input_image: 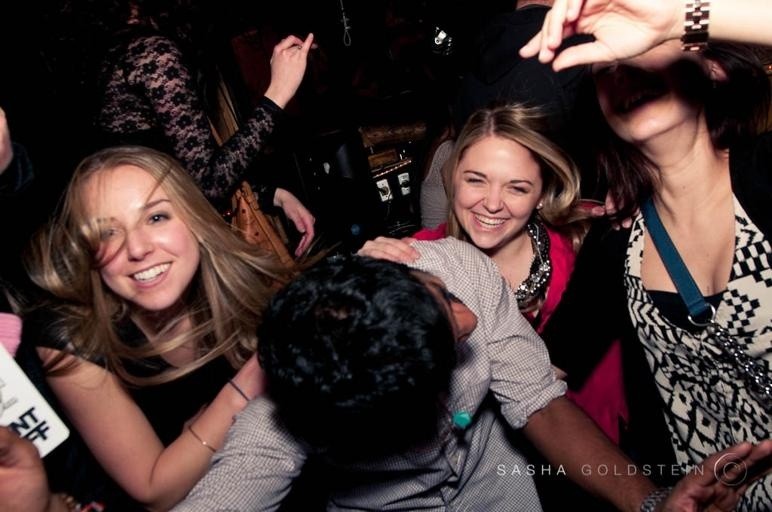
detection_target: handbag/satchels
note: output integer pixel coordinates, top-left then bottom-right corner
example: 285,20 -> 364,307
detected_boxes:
231,182 -> 295,293
